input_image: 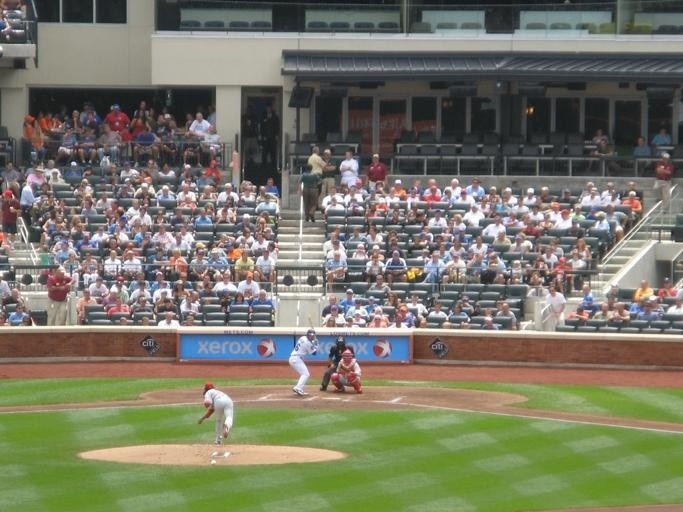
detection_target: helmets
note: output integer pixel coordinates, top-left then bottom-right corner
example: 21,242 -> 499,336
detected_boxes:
342,349 -> 352,363
336,336 -> 345,348
307,329 -> 315,336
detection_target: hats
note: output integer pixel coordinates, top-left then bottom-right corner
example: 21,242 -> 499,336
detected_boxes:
205,383 -> 213,389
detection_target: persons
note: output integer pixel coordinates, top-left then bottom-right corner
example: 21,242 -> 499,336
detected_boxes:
288,328 -> 320,395
197,382 -> 236,446
0,95 -> 280,326
300,124 -> 683,331
0,9 -> 12,43
319,335 -> 355,390
330,348 -> 363,394
0,0 -> 22,10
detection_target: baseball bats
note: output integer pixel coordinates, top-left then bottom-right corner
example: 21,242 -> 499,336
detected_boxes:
308,317 -> 317,339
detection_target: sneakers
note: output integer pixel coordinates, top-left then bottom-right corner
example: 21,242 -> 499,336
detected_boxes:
320,384 -> 327,391
214,424 -> 228,444
334,386 -> 344,392
354,387 -> 362,393
292,388 -> 304,396
